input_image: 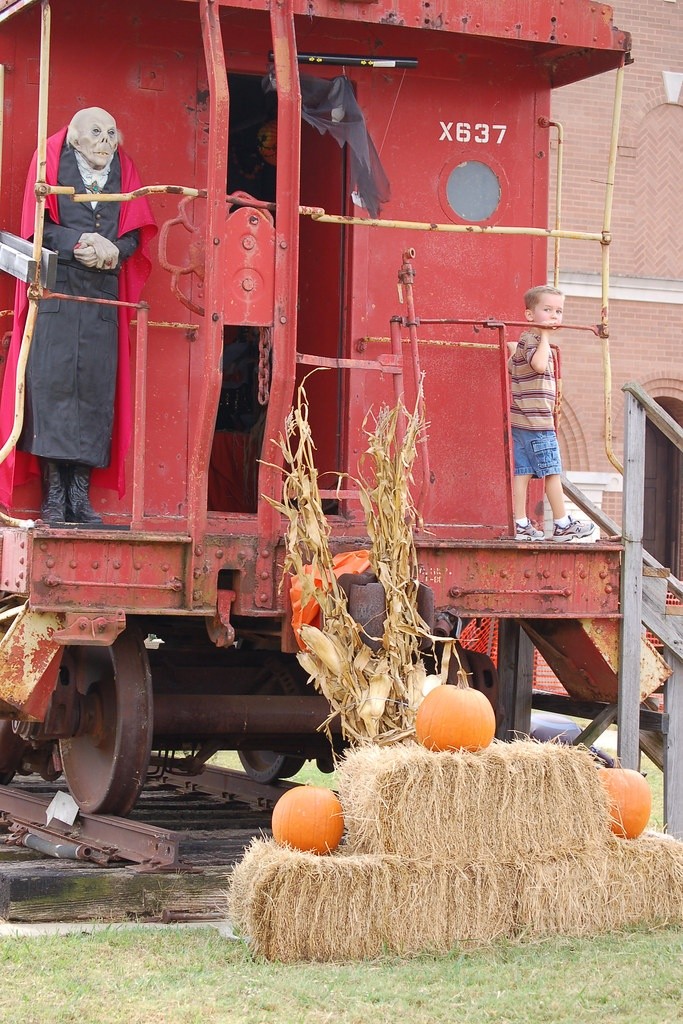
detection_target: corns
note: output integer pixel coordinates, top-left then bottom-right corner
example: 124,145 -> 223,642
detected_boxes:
296,623 -> 341,681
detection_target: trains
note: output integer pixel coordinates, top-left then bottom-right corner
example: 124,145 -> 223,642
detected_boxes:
0,1 -> 675,820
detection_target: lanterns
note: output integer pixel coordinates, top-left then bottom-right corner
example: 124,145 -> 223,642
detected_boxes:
256,121 -> 278,166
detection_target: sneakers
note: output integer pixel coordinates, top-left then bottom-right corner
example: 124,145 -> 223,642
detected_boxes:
515,519 -> 545,541
552,515 -> 595,542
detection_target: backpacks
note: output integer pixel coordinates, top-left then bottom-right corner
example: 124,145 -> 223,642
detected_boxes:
213,330 -> 263,433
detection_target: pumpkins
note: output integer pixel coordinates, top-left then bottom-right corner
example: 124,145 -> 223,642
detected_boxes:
415,672 -> 495,754
272,781 -> 344,856
596,757 -> 652,839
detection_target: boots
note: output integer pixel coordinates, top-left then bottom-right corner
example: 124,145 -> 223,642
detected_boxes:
67,462 -> 102,522
41,458 -> 68,522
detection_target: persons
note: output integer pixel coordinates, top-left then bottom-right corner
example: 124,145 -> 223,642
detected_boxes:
508,286 -> 596,540
0,106 -> 160,524
